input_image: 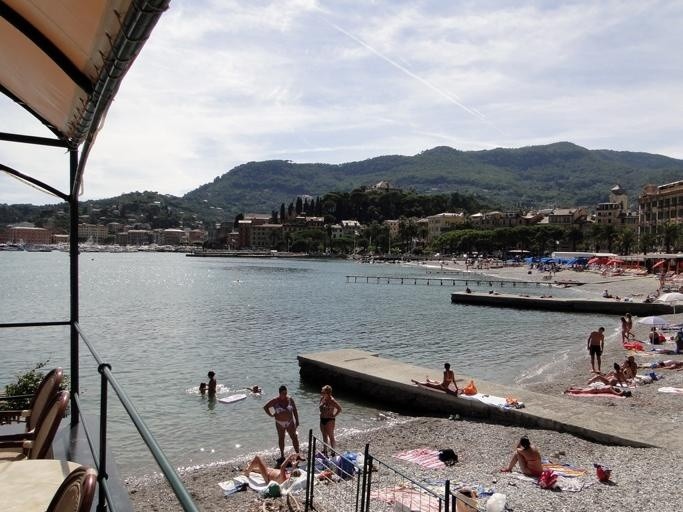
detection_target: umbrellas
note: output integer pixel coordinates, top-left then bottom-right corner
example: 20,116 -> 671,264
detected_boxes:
651,291 -> 683,319
525,256 -> 624,272
637,316 -> 670,344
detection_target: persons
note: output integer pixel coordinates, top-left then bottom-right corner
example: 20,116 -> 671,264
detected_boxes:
199,371 -> 216,399
651,289 -> 660,297
637,359 -> 683,369
620,313 -> 635,343
649,326 -> 659,344
319,385 -> 342,457
263,386 -> 305,461
250,385 -> 261,393
241,452 -> 301,485
602,289 -> 612,298
501,436 -> 542,476
563,356 -> 637,396
411,362 -> 458,393
587,327 -> 605,373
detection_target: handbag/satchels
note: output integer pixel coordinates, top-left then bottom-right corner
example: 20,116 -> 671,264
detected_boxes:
439,448 -> 458,466
537,469 -> 558,489
463,381 -> 477,395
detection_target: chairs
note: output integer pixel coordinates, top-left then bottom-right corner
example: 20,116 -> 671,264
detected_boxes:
45,463 -> 98,512
0,367 -> 71,457
